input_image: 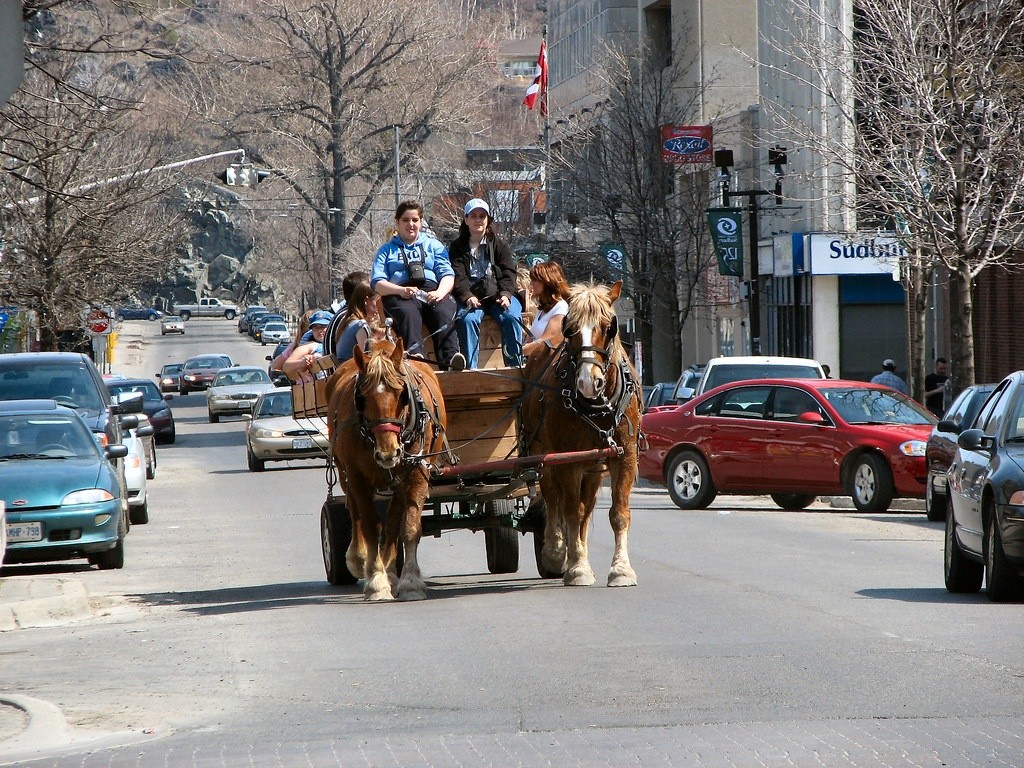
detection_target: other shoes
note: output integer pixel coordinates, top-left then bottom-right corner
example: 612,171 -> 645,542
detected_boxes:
448,353 -> 466,371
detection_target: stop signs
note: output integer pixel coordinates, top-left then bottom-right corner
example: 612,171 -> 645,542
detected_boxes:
87,309 -> 109,332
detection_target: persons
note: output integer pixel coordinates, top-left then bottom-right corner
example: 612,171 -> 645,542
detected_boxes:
449,198 -> 522,370
370,200 -> 466,370
225,375 -> 233,386
870,357 -> 952,420
522,261 -> 573,356
822,365 -> 832,379
253,372 -> 261,382
268,397 -> 284,413
271,271 -> 391,381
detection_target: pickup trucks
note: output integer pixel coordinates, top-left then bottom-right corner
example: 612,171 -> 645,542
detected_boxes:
172,296 -> 241,320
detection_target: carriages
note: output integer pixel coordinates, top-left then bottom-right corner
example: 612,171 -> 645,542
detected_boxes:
319,279 -> 641,603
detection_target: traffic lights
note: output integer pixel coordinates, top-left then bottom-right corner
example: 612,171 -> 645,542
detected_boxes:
213,168 -> 227,185
258,169 -> 270,184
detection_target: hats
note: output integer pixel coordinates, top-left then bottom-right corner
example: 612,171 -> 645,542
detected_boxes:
464,198 -> 489,216
882,358 -> 896,366
307,310 -> 335,329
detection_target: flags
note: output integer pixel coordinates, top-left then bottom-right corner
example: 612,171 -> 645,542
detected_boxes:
522,41 -> 546,119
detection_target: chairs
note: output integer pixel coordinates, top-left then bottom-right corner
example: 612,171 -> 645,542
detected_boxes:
48,376 -> 78,400
191,362 -> 199,369
223,375 -> 233,385
250,373 -> 261,382
722,404 -> 764,415
35,429 -> 75,454
211,362 -> 219,368
111,387 -> 150,400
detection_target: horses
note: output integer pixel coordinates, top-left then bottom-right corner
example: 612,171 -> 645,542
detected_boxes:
323,336 -> 448,603
524,278 -> 644,586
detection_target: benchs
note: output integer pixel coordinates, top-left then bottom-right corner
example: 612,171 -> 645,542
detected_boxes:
374,297 -> 534,328
283,354 -> 338,418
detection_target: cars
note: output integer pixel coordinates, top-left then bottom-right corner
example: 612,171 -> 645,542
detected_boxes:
161,316 -> 186,335
264,341 -> 298,386
1,352 -> 157,572
637,351 -> 1004,522
938,367 -> 1024,602
637,378 -> 940,513
242,386 -> 332,471
237,303 -> 291,346
206,366 -> 282,423
104,379 -> 176,444
155,363 -> 184,393
114,304 -> 164,321
181,355 -> 240,395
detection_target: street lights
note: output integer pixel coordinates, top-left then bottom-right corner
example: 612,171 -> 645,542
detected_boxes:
532,193 -> 651,385
715,143 -> 788,356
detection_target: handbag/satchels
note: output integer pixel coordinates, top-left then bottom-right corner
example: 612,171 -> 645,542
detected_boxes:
470,273 -> 498,306
408,261 -> 426,283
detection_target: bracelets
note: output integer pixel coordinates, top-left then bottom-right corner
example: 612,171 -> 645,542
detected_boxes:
534,339 -> 541,346
